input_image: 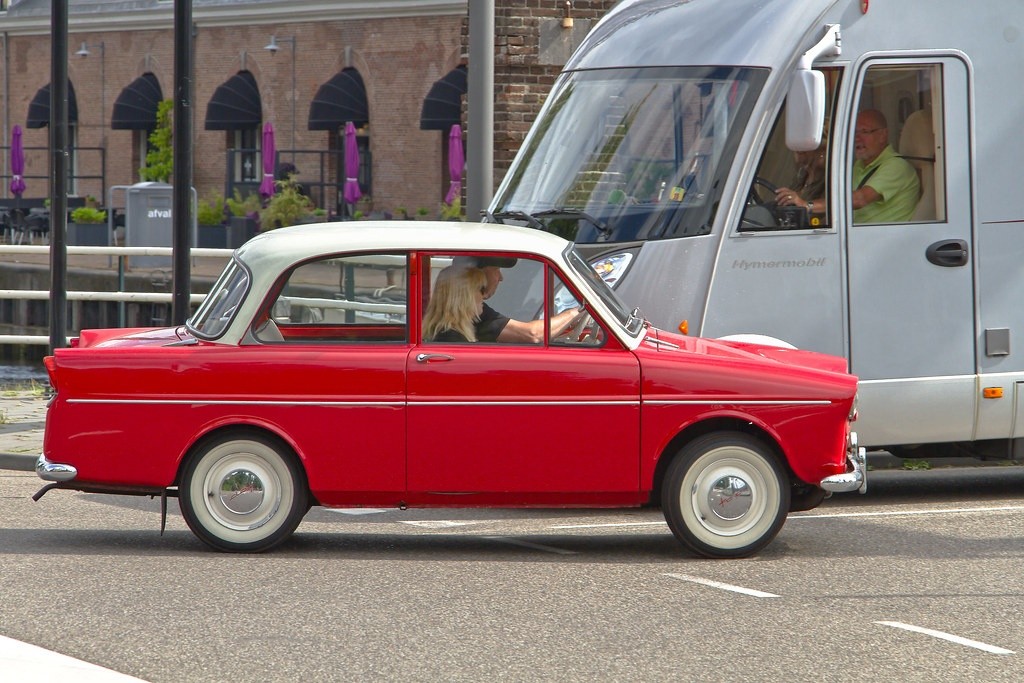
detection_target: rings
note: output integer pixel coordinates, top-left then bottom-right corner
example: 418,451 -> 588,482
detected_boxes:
787,195 -> 792,199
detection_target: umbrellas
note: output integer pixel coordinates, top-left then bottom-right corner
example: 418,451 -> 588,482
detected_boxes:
260,121 -> 274,196
10,124 -> 26,200
444,123 -> 464,206
345,120 -> 361,219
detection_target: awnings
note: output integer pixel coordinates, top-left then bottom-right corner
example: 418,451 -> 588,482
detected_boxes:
110,72 -> 164,130
418,63 -> 468,130
308,67 -> 368,131
204,70 -> 262,130
26,77 -> 78,129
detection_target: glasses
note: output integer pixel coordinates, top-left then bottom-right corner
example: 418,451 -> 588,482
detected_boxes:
480,285 -> 486,296
856,128 -> 881,134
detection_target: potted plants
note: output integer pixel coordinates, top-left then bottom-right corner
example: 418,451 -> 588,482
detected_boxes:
312,207 -> 328,223
67,206 -> 108,247
225,188 -> 263,249
354,210 -> 364,221
391,207 -> 408,220
356,195 -> 375,211
197,186 -> 231,250
414,207 -> 430,221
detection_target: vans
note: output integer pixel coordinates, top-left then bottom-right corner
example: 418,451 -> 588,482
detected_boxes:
477,0 -> 1024,463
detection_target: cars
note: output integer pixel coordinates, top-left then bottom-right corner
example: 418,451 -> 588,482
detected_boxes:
33,224 -> 867,555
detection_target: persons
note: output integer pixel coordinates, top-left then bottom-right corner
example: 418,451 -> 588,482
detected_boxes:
421,256 -> 593,344
774,110 -> 920,227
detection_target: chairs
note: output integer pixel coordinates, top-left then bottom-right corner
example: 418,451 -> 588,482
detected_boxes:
897,109 -> 937,221
0,206 -> 124,247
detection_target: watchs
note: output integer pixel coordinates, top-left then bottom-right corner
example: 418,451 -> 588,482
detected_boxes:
808,200 -> 813,214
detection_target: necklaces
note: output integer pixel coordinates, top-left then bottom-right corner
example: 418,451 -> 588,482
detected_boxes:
805,180 -> 814,187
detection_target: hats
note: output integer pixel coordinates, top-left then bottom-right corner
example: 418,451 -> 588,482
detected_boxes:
452,255 -> 517,269
